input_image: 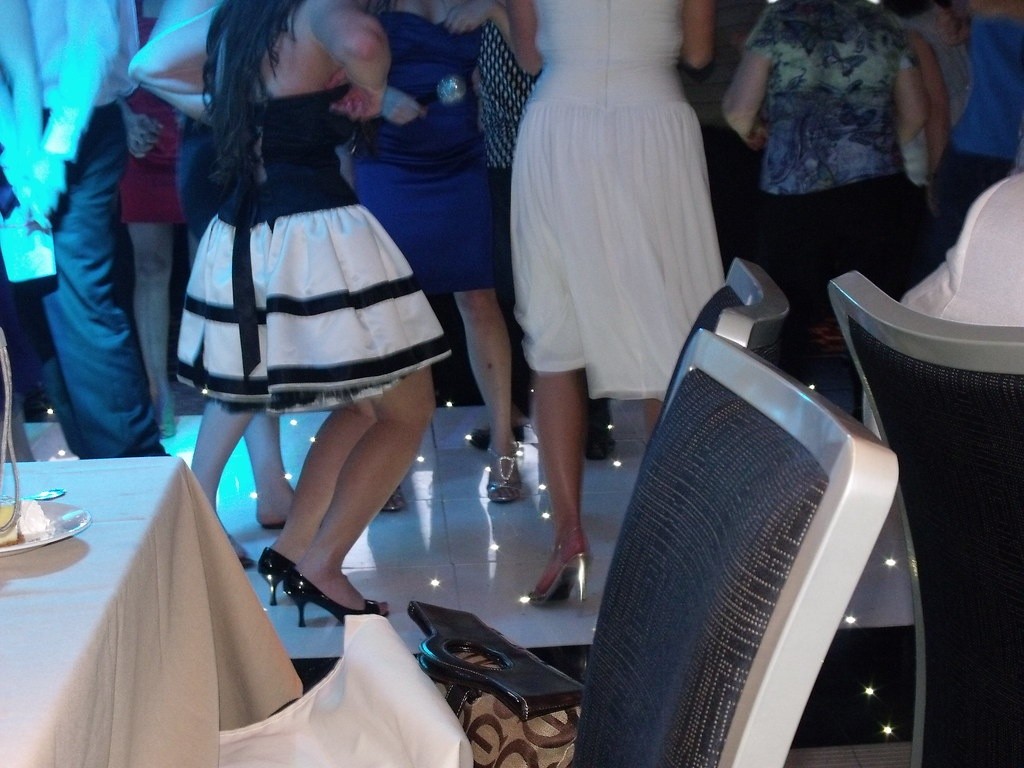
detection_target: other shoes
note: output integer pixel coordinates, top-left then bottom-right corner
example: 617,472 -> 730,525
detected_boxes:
584,425 -> 610,460
470,427 -> 526,448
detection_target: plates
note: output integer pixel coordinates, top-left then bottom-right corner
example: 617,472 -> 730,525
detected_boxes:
0,501 -> 92,557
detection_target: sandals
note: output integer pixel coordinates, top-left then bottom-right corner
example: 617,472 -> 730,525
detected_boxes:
487,443 -> 522,501
381,487 -> 404,512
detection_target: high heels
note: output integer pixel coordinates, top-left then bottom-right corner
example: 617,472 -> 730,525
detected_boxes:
257,547 -> 297,606
283,567 -> 389,628
529,525 -> 591,605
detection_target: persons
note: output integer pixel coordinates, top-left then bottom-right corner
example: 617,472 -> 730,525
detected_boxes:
116,0 -> 181,439
467,0 -> 612,460
1,0 -> 172,457
179,406 -> 292,569
128,0 -> 452,627
350,0 -> 522,504
720,0 -> 1024,422
508,1 -> 725,604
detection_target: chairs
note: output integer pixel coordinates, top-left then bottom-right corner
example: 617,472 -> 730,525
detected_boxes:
640,258 -> 790,465
829,269 -> 1024,768
409,328 -> 899,768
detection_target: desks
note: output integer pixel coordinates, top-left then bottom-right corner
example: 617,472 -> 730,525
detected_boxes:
0,454 -> 303,768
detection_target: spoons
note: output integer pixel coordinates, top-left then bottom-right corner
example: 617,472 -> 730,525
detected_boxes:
20,488 -> 66,500
